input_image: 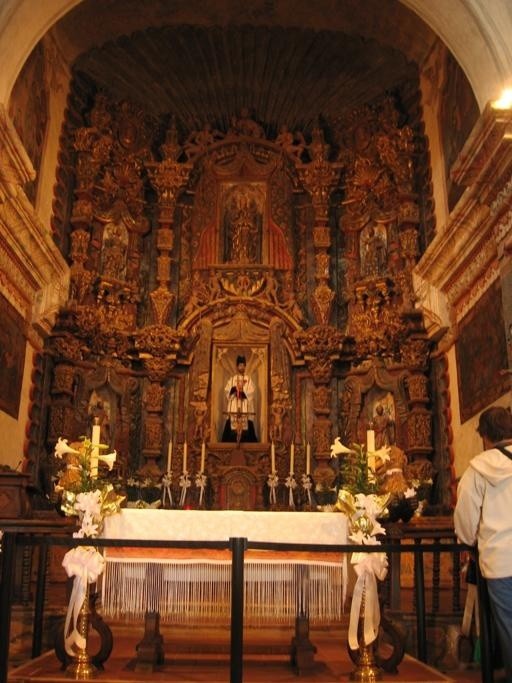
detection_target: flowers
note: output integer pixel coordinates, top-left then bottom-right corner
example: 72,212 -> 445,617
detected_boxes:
324,435 -> 435,497
47,431 -> 128,492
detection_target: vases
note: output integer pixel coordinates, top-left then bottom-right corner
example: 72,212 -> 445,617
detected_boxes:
336,489 -> 390,681
59,482 -> 126,682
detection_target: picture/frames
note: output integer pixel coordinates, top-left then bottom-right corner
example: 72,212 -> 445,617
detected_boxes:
208,338 -> 272,450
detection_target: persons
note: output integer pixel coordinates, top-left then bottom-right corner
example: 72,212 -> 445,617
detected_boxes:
454,406 -> 512,683
182,118 -> 303,159
102,228 -> 128,278
221,354 -> 258,443
194,408 -> 207,438
271,405 -> 286,438
373,405 -> 389,448
85,401 -> 110,444
363,226 -> 384,277
176,269 -> 299,324
224,194 -> 257,262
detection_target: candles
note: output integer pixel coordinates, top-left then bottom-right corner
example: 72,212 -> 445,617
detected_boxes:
167,438 -> 173,474
200,440 -> 206,475
182,438 -> 188,473
91,425 -> 100,485
271,439 -> 277,474
289,440 -> 294,475
305,440 -> 311,476
366,429 -> 377,485
